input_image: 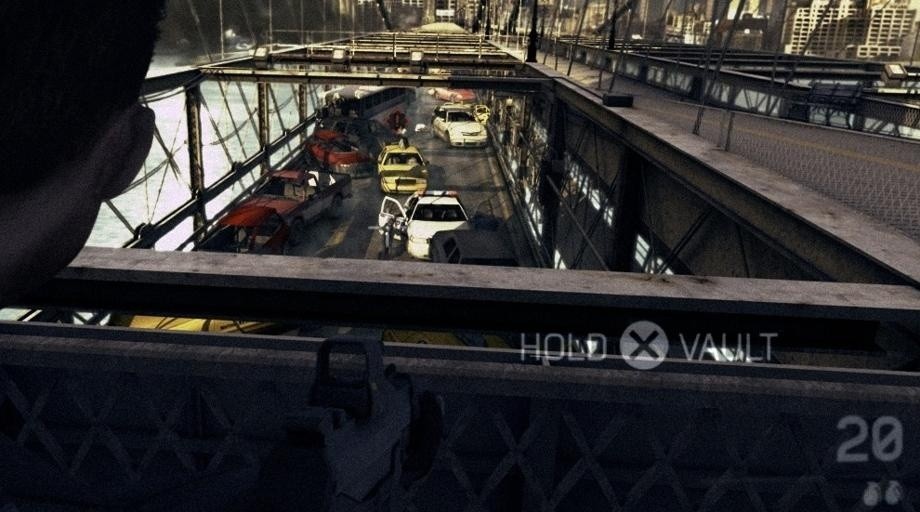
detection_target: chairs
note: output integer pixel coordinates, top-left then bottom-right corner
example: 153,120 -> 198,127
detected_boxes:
442,209 -> 457,219
386,158 -> 397,164
406,157 -> 417,165
421,209 -> 433,219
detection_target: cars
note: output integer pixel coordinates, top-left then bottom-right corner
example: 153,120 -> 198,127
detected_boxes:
378,138 -> 429,194
378,189 -> 474,261
430,88 -> 490,151
127,312 -> 274,336
428,229 -> 520,266
189,206 -> 292,256
307,116 -> 398,178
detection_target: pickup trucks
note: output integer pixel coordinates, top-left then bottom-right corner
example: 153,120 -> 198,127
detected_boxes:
239,169 -> 354,236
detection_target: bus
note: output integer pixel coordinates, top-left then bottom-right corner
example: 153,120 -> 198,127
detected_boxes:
315,86 -> 418,135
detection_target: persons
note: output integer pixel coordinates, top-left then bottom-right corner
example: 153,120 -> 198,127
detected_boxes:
396,124 -> 408,148
377,215 -> 395,259
1,0 -> 169,307
